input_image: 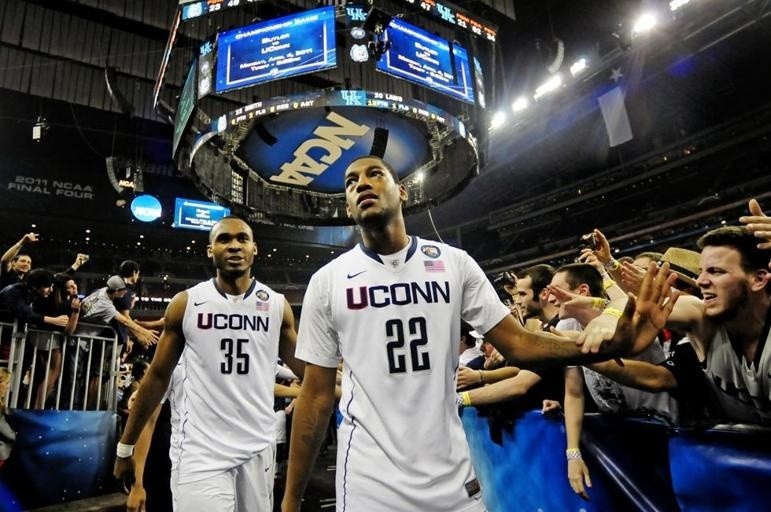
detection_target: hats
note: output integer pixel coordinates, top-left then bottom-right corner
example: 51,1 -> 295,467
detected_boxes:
644,246 -> 701,287
106,275 -> 134,290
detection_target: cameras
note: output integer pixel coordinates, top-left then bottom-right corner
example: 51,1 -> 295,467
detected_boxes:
493,271 -> 515,286
71,295 -> 99,313
578,233 -> 595,250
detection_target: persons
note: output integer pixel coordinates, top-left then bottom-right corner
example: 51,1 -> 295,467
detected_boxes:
114,217 -> 305,512
456,155 -> 770,507
0,232 -> 164,417
275,357 -> 344,490
283,155 -> 679,512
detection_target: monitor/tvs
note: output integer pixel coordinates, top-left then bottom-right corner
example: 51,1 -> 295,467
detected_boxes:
172,197 -> 231,233
